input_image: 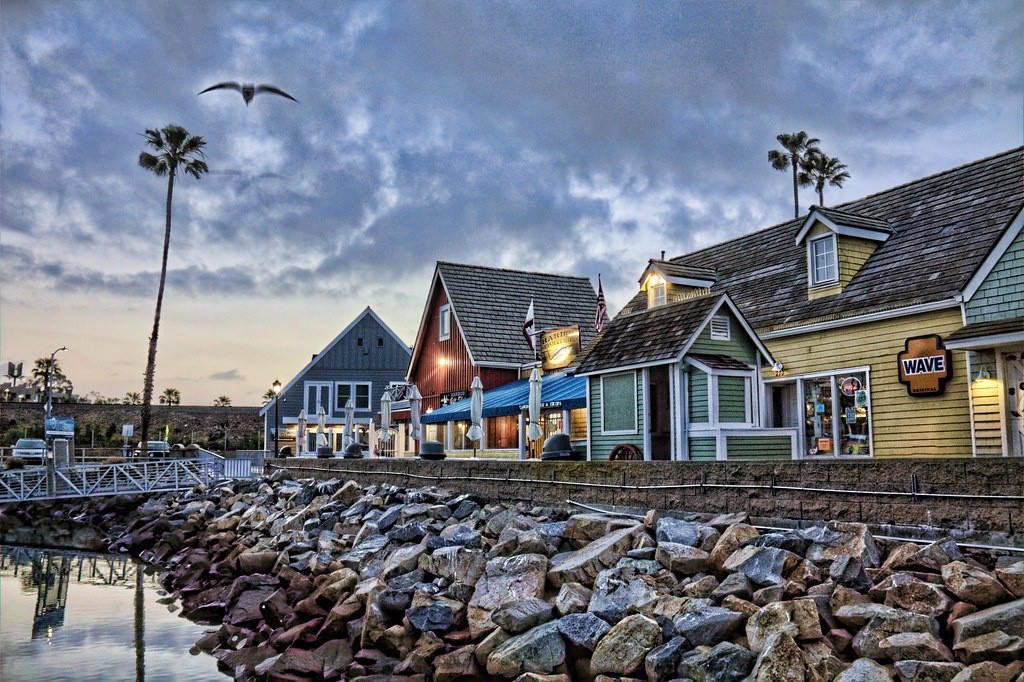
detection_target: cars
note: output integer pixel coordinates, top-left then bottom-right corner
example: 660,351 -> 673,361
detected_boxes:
11,438 -> 48,465
134,440 -> 172,466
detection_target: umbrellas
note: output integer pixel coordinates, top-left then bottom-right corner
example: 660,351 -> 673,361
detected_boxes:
527,364 -> 544,459
376,427 -> 397,454
343,399 -> 355,455
296,409 -> 307,457
407,385 -> 424,458
377,391 -> 391,458
466,375 -> 485,458
316,407 -> 327,455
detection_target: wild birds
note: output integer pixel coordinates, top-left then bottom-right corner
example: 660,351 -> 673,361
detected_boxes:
196,79 -> 303,108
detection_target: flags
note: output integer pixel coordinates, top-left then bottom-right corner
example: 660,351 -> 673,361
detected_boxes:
522,299 -> 536,360
593,277 -> 607,333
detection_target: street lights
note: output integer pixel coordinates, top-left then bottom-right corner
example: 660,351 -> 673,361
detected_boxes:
46,346 -> 69,417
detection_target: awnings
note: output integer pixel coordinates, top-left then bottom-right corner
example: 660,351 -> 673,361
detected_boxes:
419,372 -> 586,424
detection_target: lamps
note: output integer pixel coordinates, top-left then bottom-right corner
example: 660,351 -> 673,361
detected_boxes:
974,351 -> 990,381
